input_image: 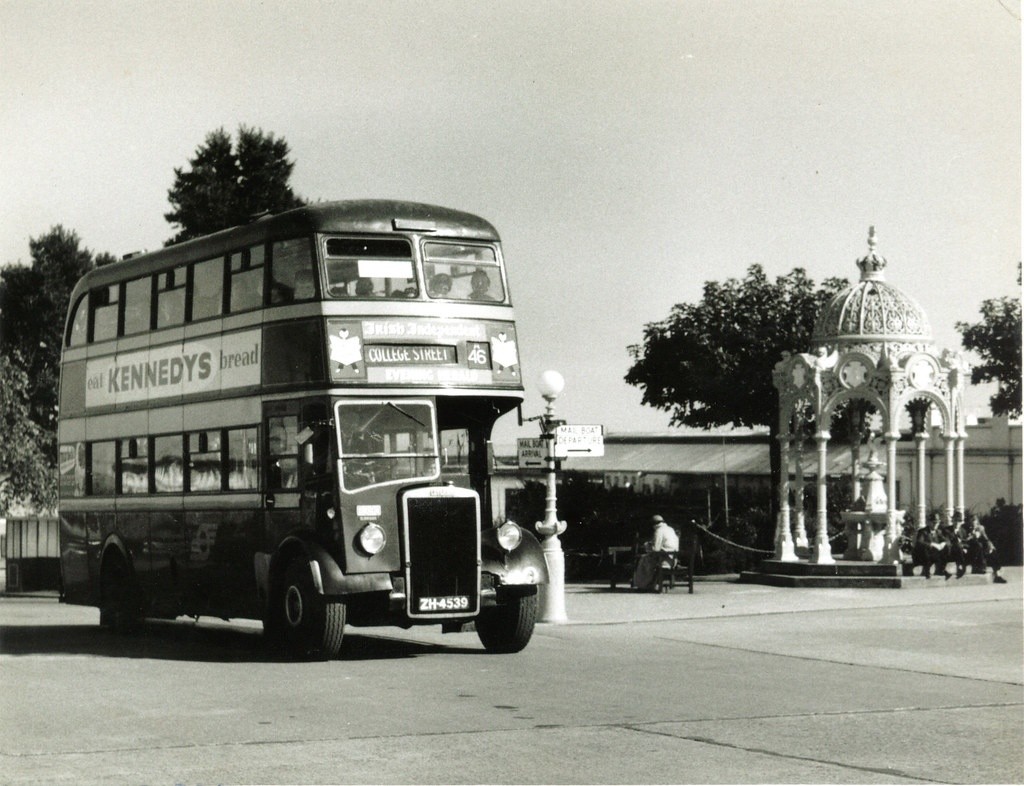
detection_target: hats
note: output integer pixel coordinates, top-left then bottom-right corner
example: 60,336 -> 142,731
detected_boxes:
650,515 -> 665,526
928,512 -> 941,523
951,511 -> 962,522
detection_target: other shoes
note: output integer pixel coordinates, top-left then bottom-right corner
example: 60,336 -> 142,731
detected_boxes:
935,570 -> 949,575
973,567 -> 986,574
993,576 -> 1006,583
958,569 -> 964,575
922,570 -> 930,576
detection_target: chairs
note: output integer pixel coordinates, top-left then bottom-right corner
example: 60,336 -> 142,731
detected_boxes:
650,550 -> 696,594
607,546 -> 633,590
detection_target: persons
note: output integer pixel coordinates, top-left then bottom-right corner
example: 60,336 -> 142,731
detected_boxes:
956,515 -> 1008,583
913,512 -> 965,579
628,511 -> 681,592
